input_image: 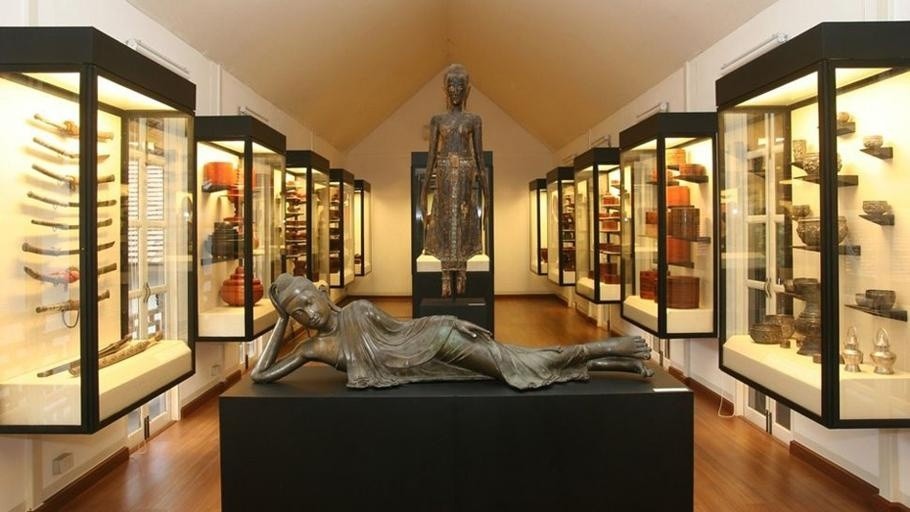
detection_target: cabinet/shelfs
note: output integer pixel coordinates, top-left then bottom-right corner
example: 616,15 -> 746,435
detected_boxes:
196,116 -> 286,343
284,150 -> 330,297
353,179 -> 371,277
620,112 -> 717,338
411,152 -> 494,340
330,169 -> 355,289
529,178 -> 547,276
546,166 -> 575,287
1,25 -> 197,435
574,148 -> 621,304
218,366 -> 694,511
715,21 -> 910,429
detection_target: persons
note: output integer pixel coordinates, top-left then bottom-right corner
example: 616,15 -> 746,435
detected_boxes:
419,63 -> 491,297
250,272 -> 656,390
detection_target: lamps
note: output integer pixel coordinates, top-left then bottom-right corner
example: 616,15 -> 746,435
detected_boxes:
240,106 -> 269,127
584,135 -> 610,151
127,37 -> 190,79
720,32 -> 786,77
636,102 -> 668,125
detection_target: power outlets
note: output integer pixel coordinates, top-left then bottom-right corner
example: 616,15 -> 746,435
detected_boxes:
52,453 -> 73,476
